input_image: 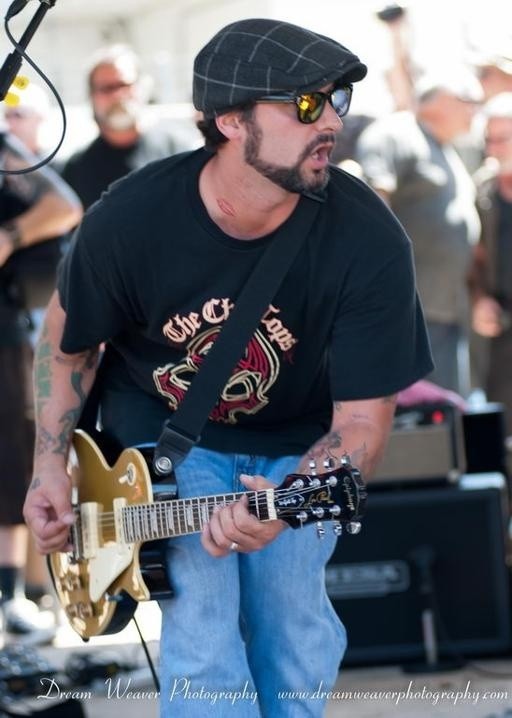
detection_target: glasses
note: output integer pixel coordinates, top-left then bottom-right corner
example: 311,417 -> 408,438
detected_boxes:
257,84 -> 353,123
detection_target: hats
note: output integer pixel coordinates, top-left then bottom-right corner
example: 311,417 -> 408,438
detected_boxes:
193,19 -> 367,119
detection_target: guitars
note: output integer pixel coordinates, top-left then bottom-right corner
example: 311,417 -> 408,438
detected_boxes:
47,426 -> 368,642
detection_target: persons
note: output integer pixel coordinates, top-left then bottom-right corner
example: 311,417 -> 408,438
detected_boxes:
0,1 -> 512,718
